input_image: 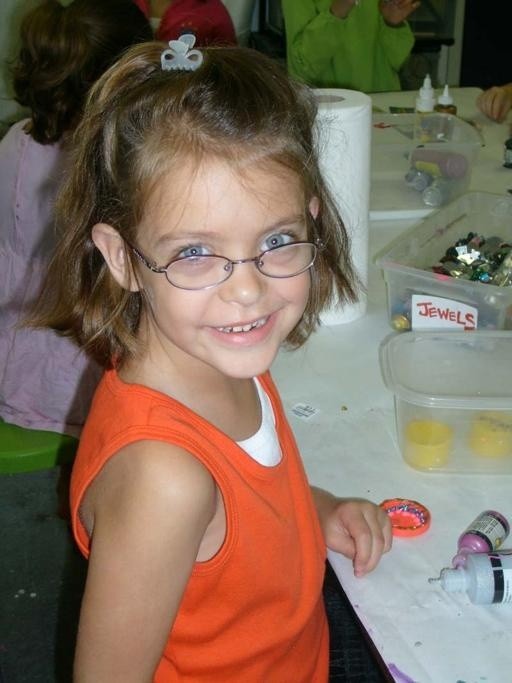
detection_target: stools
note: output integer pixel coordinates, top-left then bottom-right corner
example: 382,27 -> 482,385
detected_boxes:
0,416 -> 79,480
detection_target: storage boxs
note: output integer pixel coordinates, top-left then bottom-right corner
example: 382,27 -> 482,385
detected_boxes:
374,190 -> 511,329
380,333 -> 509,476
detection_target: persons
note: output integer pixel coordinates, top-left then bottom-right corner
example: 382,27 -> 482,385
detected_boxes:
147,0 -> 266,52
2,1 -> 156,439
278,1 -> 430,97
23,33 -> 393,680
478,82 -> 508,120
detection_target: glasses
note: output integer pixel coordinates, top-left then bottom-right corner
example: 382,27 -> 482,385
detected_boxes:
119,209 -> 327,290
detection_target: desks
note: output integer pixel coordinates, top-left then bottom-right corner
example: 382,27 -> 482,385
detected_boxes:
260,69 -> 509,683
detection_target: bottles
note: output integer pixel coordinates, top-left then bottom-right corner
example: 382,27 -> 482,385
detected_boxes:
449,508 -> 508,568
426,550 -> 512,607
412,75 -> 458,142
